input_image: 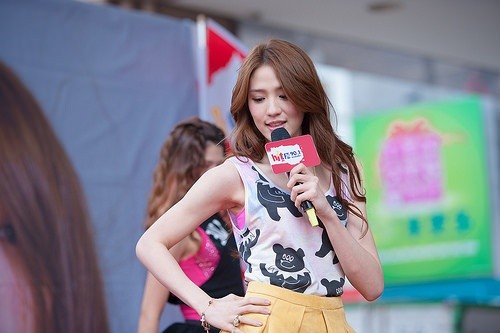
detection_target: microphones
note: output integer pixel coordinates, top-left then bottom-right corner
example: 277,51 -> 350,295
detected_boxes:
264,127 -> 320,227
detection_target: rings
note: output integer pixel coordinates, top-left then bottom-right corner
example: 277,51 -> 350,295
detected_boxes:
233,315 -> 240,327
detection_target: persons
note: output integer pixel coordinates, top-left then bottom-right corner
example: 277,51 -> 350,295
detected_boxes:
0,63 -> 110,333
137,120 -> 246,333
136,39 -> 384,333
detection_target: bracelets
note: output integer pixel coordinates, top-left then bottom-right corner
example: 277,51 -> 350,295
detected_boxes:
200,298 -> 214,333
